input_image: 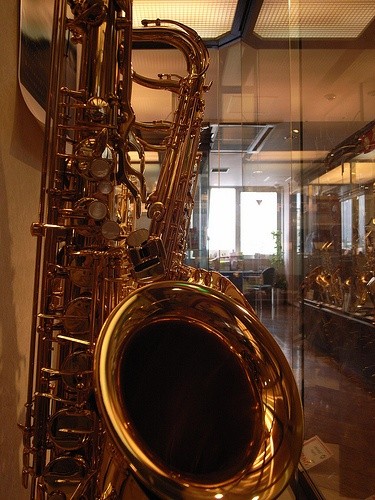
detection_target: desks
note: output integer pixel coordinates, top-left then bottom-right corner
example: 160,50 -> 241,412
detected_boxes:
211,268 -> 262,311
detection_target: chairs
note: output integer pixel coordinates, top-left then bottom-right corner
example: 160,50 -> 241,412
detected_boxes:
253,266 -> 278,318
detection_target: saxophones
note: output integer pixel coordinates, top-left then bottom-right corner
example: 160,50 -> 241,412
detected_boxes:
15,0 -> 304,500
317,226 -> 375,304
